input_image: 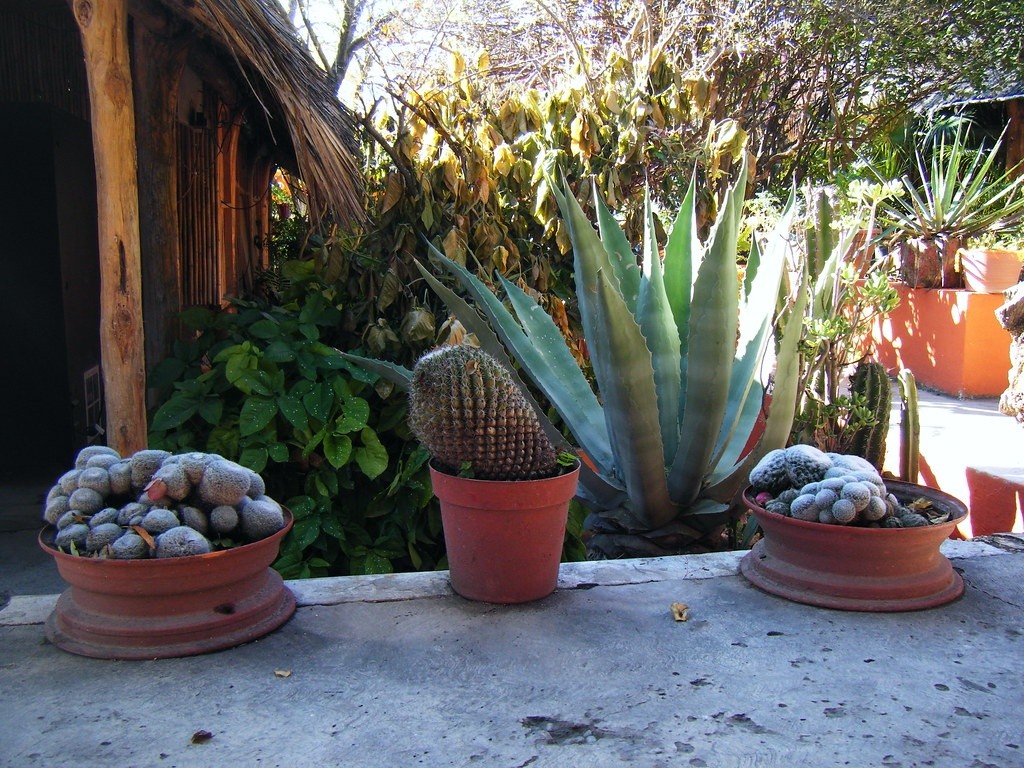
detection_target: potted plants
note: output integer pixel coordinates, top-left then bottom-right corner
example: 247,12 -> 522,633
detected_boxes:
955,232 -> 1024,290
37,443 -> 298,660
833,181 -> 904,278
741,447 -> 971,613
845,114 -> 1024,288
407,348 -> 583,601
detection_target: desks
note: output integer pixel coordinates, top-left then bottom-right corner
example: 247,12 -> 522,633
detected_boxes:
845,281 -> 1013,400
